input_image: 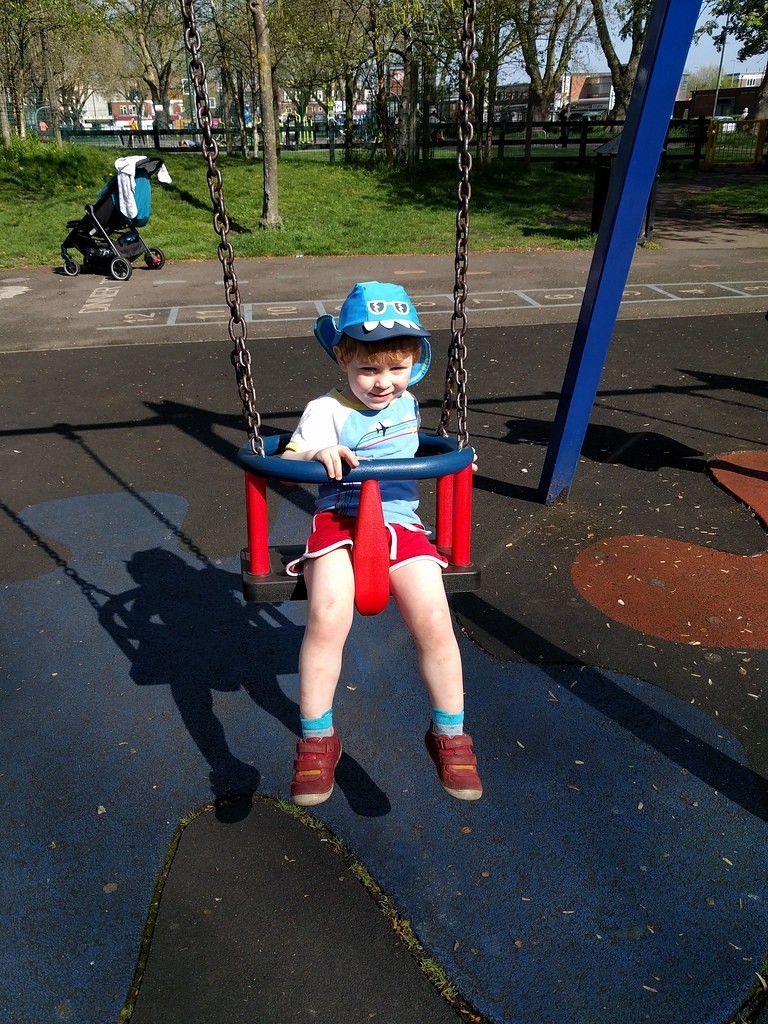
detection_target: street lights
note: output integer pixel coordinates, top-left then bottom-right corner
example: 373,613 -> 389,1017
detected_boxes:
731,59 -> 736,88
180,40 -> 196,142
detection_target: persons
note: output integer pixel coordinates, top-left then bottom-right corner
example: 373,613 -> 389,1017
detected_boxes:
276,282 -> 482,805
39,120 -> 48,140
179,140 -> 207,147
127,118 -> 137,147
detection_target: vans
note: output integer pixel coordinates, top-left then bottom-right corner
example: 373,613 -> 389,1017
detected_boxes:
708,116 -> 736,134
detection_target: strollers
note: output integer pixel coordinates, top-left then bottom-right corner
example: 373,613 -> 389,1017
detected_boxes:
60,156 -> 164,281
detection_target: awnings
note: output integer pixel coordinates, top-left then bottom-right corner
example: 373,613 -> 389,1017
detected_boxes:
582,110 -> 606,116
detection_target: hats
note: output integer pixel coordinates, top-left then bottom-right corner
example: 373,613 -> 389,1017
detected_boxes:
311,280 -> 432,387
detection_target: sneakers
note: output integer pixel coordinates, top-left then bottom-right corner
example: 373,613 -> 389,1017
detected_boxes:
290,722 -> 343,805
424,716 -> 483,801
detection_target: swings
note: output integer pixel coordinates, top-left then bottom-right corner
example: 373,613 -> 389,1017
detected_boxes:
178,0 -> 481,605
